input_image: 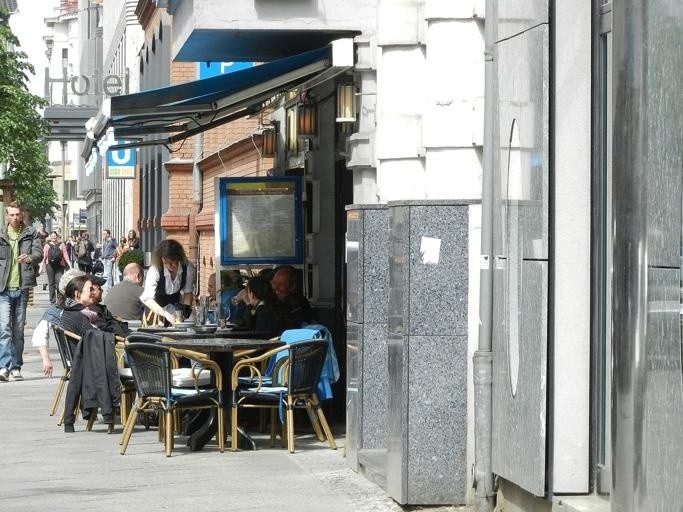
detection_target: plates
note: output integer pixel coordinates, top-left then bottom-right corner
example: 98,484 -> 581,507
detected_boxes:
175,322 -> 195,328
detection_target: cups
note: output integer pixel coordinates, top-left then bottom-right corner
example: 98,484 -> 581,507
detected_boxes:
176,296 -> 234,327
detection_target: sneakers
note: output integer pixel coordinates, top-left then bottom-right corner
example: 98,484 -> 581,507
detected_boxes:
10,369 -> 24,380
0,367 -> 10,381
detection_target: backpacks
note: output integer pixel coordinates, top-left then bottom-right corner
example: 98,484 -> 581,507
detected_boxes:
46,242 -> 62,265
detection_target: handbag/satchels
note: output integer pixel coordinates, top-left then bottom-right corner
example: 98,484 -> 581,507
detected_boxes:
78,244 -> 86,258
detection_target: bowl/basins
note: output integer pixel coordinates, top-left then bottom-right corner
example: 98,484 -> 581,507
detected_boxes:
192,325 -> 218,334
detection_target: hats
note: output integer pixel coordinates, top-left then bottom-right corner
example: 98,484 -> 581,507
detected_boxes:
87,274 -> 106,285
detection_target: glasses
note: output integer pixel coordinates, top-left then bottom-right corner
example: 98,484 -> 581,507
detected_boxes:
81,287 -> 94,292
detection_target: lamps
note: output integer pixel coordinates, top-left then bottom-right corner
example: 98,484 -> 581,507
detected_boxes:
260,129 -> 278,158
335,79 -> 357,123
297,96 -> 317,140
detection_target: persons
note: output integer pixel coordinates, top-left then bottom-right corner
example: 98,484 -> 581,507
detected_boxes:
31,264 -> 314,380
36,220 -> 141,306
105,262 -> 145,320
0,202 -> 45,382
138,239 -> 197,329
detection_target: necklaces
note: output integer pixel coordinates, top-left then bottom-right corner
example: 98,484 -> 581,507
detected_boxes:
7,223 -> 23,241
